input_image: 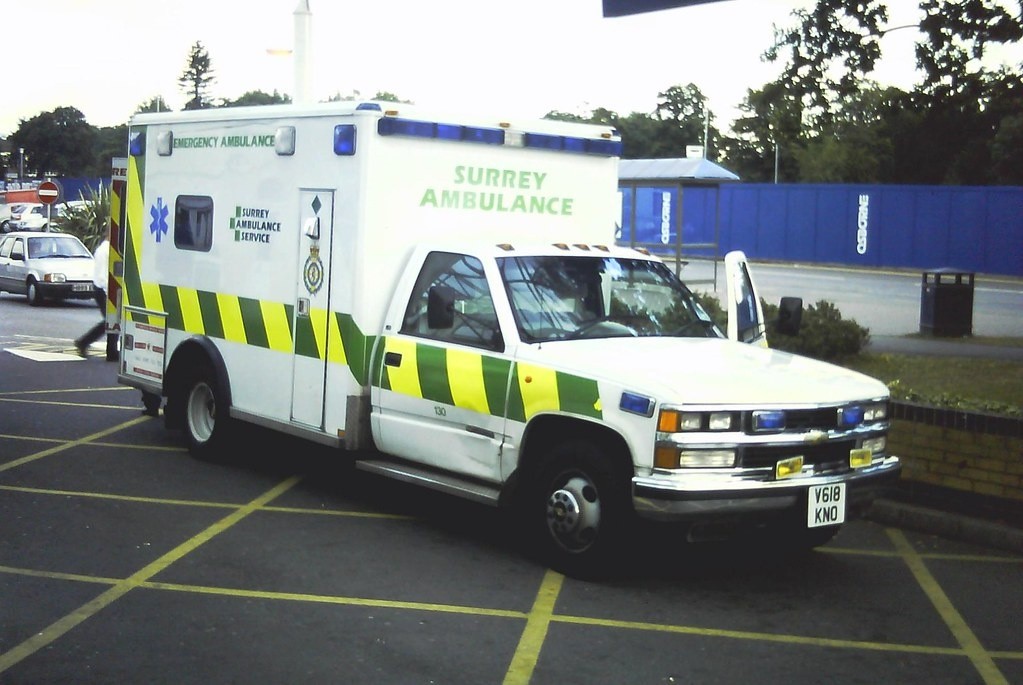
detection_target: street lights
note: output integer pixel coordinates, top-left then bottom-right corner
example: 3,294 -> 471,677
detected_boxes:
19,146 -> 24,190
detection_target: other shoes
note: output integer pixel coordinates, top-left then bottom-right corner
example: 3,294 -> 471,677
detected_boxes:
74,340 -> 86,357
106,350 -> 120,361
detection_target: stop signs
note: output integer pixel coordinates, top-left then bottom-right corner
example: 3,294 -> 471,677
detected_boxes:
37,182 -> 59,205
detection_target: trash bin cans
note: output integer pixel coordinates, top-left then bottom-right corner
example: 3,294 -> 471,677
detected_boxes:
919,266 -> 975,338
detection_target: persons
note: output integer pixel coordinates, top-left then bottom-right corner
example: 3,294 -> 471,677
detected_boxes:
74,224 -> 119,362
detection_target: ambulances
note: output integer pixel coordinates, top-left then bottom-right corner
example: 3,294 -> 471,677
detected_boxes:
103,101 -> 906,586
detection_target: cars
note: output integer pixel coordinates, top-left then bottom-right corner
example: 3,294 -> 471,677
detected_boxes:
0,232 -> 96,307
0,200 -> 92,233
